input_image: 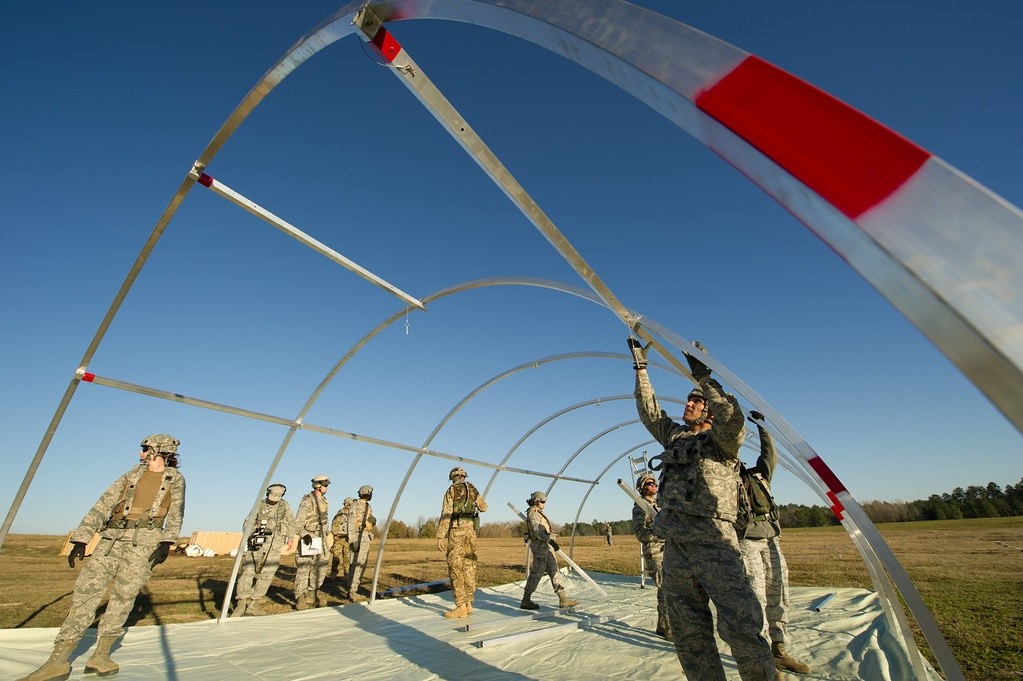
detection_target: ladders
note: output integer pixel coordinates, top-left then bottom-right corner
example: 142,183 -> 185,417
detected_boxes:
628,450 -> 656,589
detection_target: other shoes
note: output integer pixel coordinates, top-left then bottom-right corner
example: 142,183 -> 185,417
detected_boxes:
656,629 -> 674,642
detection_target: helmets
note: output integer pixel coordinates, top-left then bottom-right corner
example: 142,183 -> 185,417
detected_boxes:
636,475 -> 656,494
688,387 -> 705,398
531,491 -> 547,502
449,467 -> 467,480
266,476 -> 373,507
140,434 -> 180,453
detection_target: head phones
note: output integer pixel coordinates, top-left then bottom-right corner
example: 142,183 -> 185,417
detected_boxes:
266,484 -> 286,496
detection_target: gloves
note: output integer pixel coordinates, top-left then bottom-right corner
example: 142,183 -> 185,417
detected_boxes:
549,539 -> 559,551
68,543 -> 85,568
627,335 -> 653,365
682,340 -> 711,380
747,410 -> 765,428
148,542 -> 170,567
303,516 -> 376,552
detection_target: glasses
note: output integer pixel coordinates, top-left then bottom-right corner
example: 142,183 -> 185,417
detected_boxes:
540,501 -> 546,503
142,447 -> 149,452
645,482 -> 654,486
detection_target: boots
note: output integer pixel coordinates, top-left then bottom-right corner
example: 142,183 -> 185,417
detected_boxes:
230,589 -> 365,617
444,605 -> 473,618
771,641 -> 811,674
557,591 -> 576,608
521,596 -> 539,610
83,636 -> 119,676
15,643 -> 77,681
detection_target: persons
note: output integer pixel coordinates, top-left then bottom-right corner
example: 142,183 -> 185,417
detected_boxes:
628,335 -> 812,681
229,484 -> 296,619
15,435 -> 186,681
632,473 -> 673,643
330,485 -> 377,599
604,521 -> 612,545
295,475 -> 332,610
436,467 -> 488,619
520,491 -> 577,610
